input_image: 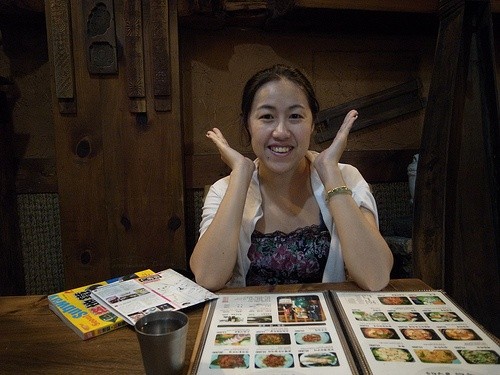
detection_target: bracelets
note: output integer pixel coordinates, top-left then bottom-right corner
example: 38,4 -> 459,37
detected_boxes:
325,185 -> 352,208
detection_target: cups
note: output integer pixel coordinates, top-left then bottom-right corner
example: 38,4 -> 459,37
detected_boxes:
134,311 -> 189,375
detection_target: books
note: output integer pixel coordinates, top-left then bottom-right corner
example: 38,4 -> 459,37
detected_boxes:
190,289 -> 500,375
89,267 -> 219,329
47,269 -> 157,340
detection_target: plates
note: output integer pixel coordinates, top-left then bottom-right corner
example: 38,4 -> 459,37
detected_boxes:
255,332 -> 336,368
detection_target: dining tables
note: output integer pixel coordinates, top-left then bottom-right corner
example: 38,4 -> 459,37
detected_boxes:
0,277 -> 500,374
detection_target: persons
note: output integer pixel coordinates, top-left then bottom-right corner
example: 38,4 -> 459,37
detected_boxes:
188,65 -> 394,292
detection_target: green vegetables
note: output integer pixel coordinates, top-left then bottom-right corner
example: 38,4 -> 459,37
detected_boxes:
461,350 -> 499,364
